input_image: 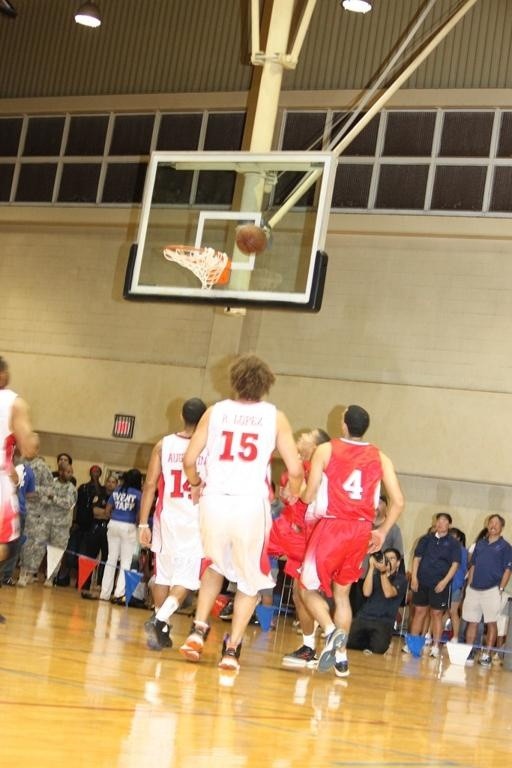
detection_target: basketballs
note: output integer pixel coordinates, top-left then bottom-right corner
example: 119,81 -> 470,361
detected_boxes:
235,226 -> 265,252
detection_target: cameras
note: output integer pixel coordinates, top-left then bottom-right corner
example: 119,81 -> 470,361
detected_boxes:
373,549 -> 392,574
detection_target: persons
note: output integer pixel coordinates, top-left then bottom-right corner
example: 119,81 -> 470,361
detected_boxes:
219,482 -> 511,667
181,350 -> 304,669
268,426 -> 330,664
1,355 -> 40,623
298,402 -> 403,678
1,444 -> 157,607
138,394 -> 207,651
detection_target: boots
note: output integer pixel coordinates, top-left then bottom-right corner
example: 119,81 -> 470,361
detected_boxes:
5,567 -> 56,589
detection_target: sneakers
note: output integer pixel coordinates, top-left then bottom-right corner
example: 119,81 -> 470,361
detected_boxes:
143,611 -> 174,652
217,632 -> 244,671
279,627 -> 351,678
179,619 -> 211,663
0,615 -> 8,625
291,616 -> 327,640
400,631 -> 505,668
